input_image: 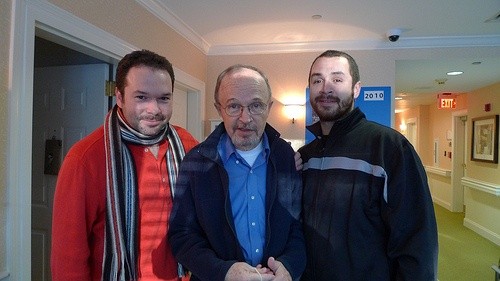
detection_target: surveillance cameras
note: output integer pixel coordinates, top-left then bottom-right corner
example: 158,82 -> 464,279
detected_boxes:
388,35 -> 399,42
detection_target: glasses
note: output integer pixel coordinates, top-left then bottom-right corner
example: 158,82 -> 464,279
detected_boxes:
218,102 -> 271,116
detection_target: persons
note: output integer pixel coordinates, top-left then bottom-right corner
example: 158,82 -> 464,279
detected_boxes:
167,64 -> 307,281
50,50 -> 303,281
297,50 -> 439,281
